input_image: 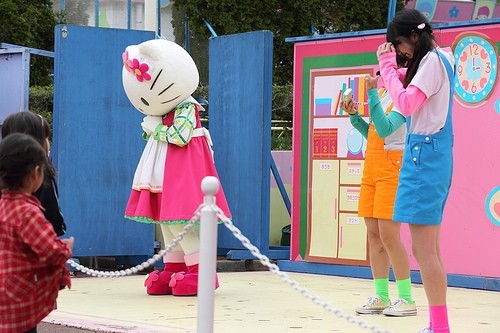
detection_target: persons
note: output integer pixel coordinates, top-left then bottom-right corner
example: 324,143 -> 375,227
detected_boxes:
118,38 -> 224,296
339,59 -> 417,318
376,8 -> 458,333
0,111 -> 65,236
2,132 -> 76,333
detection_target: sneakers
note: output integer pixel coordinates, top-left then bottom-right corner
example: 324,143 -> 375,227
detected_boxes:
383,299 -> 417,316
355,295 -> 391,314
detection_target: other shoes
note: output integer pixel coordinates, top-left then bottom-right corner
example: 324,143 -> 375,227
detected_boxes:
418,328 -> 434,333
